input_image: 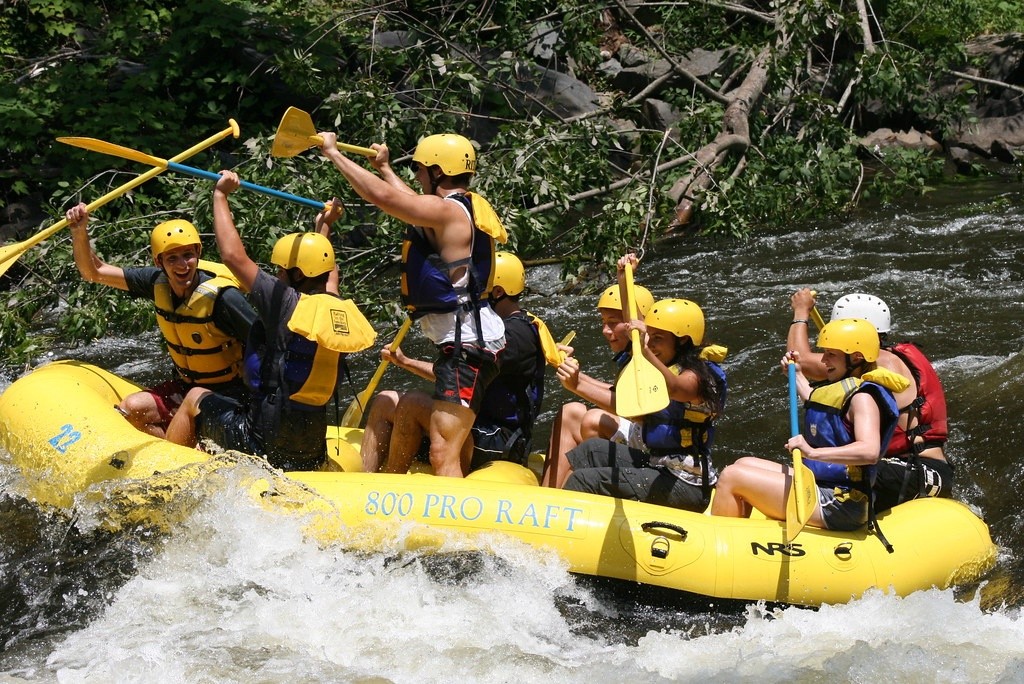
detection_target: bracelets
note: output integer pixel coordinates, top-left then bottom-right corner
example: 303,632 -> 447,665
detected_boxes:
789,320 -> 808,326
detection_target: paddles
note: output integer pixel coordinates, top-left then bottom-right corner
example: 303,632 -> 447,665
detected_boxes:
271,105 -> 377,164
340,319 -> 413,428
0,118 -> 240,278
616,258 -> 671,418
545,330 -> 577,364
55,135 -> 343,214
785,351 -> 818,542
808,305 -> 825,331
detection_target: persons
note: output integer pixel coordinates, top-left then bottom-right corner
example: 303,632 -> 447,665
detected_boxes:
358,250 -> 562,479
540,284 -> 655,489
319,130 -> 508,477
165,170 -> 375,460
558,253 -> 717,515
708,320 -> 910,537
66,202 -> 259,441
787,288 -> 954,514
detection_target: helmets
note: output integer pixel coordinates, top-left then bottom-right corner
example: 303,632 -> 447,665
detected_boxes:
830,293 -> 891,332
150,219 -> 203,266
270,231 -> 335,275
597,284 -> 653,318
644,299 -> 706,346
817,319 -> 879,363
484,250 -> 526,297
413,134 -> 476,175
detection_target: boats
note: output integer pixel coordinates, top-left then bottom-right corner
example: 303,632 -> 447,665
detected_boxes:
0,358 -> 997,613
